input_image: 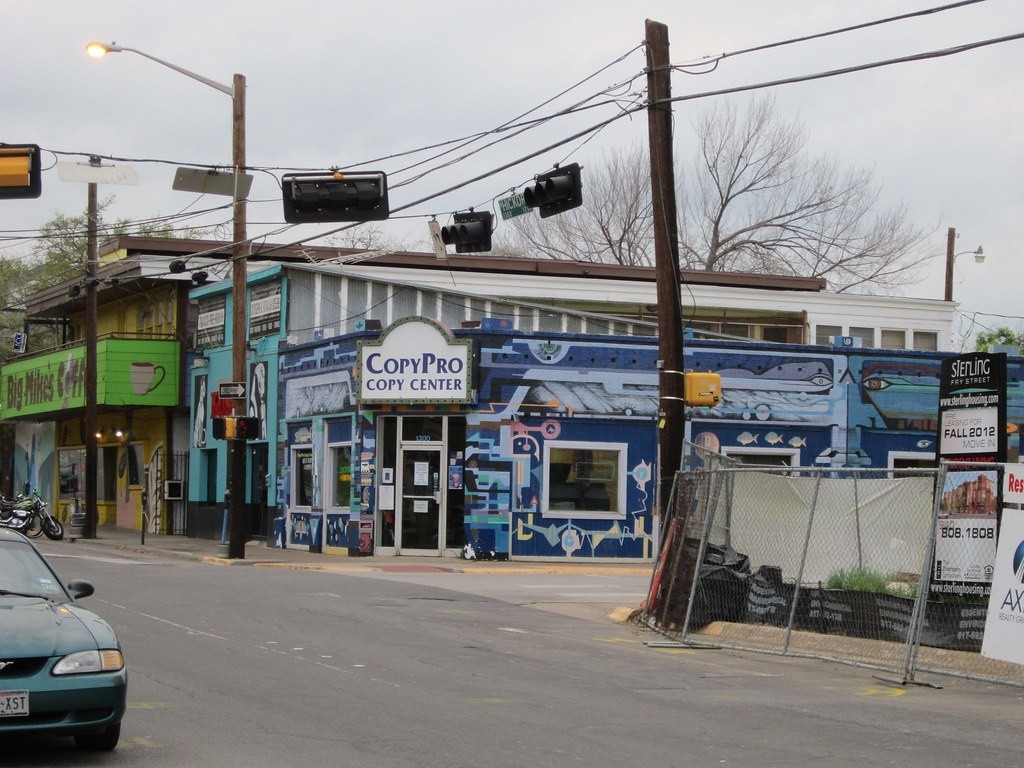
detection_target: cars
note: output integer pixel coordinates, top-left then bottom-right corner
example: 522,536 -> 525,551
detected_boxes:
0,521 -> 128,761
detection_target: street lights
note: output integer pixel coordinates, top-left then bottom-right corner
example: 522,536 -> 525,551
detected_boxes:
82,39 -> 254,414
941,226 -> 986,302
139,441 -> 164,548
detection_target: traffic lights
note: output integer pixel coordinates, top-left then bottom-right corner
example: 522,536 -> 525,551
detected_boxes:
444,211 -> 492,254
524,165 -> 583,219
212,417 -> 262,440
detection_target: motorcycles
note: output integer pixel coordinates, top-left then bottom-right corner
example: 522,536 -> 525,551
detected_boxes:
0,488 -> 64,540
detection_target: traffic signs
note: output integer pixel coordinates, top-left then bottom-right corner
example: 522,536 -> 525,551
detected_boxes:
498,194 -> 533,219
